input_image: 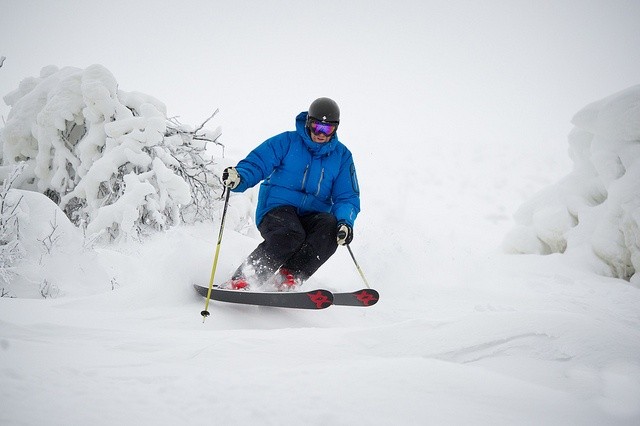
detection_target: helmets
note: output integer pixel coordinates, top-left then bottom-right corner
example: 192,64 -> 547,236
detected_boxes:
307,98 -> 340,135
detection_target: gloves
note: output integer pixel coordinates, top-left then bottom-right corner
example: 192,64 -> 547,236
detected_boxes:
337,223 -> 353,246
222,167 -> 241,189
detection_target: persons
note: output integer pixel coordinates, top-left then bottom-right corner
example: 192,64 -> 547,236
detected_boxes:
218,97 -> 361,292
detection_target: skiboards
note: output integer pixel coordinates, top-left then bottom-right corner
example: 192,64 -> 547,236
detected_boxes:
193,284 -> 379,310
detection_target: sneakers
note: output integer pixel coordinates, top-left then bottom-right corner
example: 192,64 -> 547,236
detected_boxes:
218,276 -> 251,291
264,269 -> 300,292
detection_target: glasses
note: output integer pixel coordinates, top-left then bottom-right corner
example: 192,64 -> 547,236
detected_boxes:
307,116 -> 338,137
